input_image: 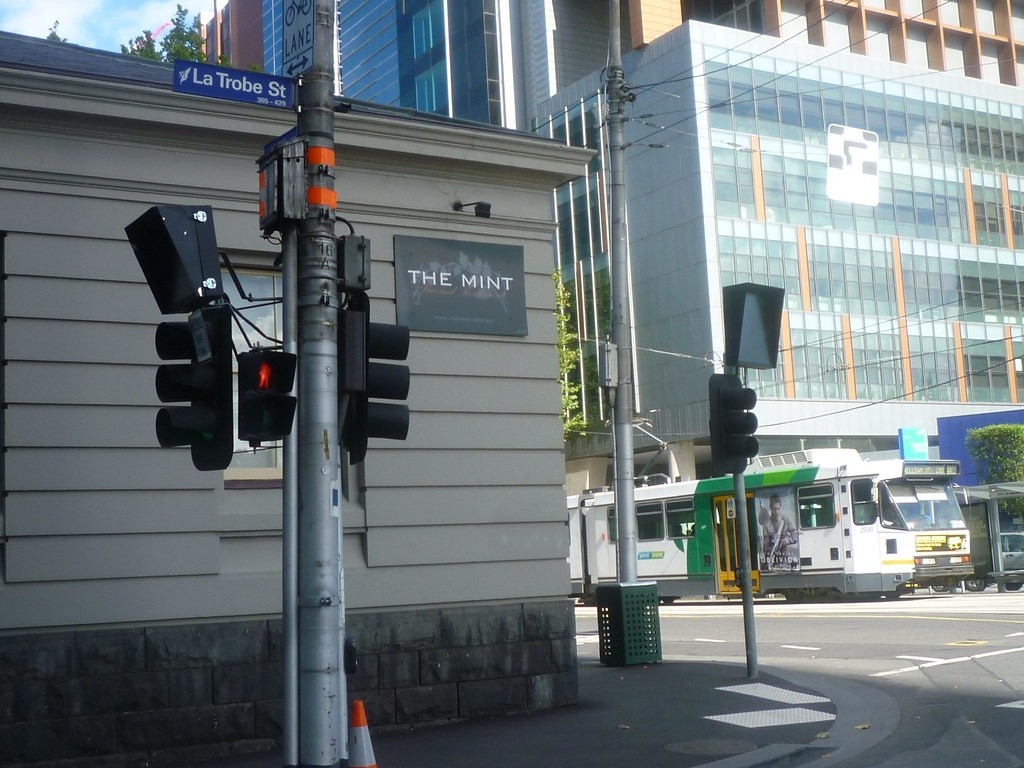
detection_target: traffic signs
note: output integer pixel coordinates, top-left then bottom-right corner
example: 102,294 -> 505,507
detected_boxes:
171,58 -> 294,111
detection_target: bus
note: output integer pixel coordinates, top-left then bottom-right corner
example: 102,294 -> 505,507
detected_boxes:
566,445 -> 975,607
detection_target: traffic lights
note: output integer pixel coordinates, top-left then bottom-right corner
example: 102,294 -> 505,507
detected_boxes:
239,350 -> 298,445
710,376 -> 760,476
124,204 -> 224,315
155,304 -> 234,471
343,291 -> 410,465
724,282 -> 785,369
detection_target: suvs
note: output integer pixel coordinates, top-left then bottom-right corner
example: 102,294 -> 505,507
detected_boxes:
965,530 -> 1024,592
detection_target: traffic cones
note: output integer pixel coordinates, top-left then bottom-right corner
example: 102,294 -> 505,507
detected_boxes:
346,698 -> 377,768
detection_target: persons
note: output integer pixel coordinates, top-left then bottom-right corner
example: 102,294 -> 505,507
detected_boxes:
691,525 -> 695,535
763,495 -> 797,565
673,525 -> 684,536
